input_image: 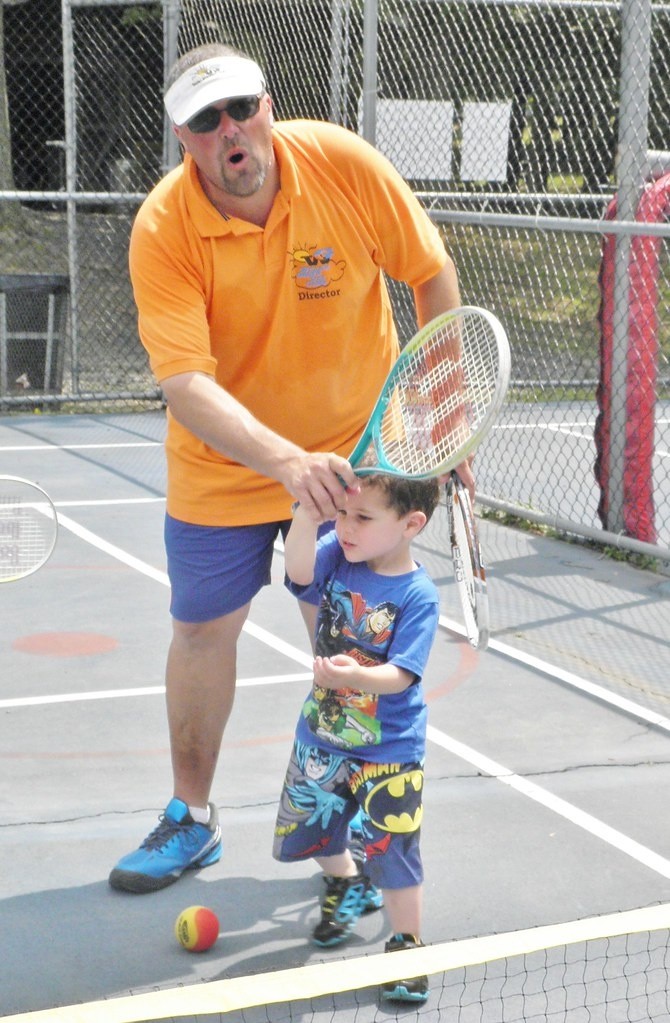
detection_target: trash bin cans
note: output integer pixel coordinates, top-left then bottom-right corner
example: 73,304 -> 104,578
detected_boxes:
0,275 -> 69,411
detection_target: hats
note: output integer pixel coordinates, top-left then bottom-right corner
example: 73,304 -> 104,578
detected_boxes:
161,57 -> 266,126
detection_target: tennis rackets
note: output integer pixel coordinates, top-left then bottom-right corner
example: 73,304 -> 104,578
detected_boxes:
0,473 -> 59,581
442,469 -> 492,653
288,304 -> 514,520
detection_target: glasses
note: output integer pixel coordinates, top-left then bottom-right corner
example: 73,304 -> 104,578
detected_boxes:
188,90 -> 266,133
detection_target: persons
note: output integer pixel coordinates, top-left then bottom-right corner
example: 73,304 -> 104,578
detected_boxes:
108,41 -> 484,902
282,451 -> 440,1003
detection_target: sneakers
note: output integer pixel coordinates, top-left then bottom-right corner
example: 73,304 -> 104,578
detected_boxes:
342,832 -> 386,912
380,932 -> 430,1001
312,859 -> 371,948
107,799 -> 222,892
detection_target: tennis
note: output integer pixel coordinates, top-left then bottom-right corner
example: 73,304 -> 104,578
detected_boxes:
175,905 -> 221,954
